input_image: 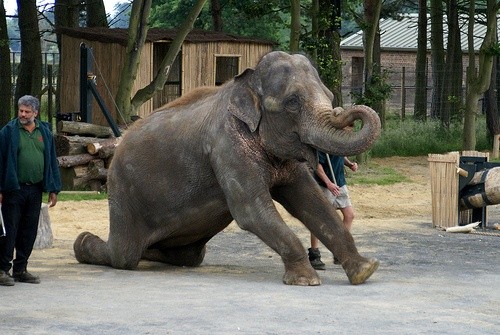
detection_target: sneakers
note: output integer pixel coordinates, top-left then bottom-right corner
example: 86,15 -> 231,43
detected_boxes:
0,272 -> 15,286
310,257 -> 327,271
15,271 -> 41,284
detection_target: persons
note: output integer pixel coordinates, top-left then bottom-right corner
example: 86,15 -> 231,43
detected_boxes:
0,95 -> 62,286
308,151 -> 358,270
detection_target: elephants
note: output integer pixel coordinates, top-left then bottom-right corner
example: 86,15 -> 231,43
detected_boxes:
73,50 -> 382,285
